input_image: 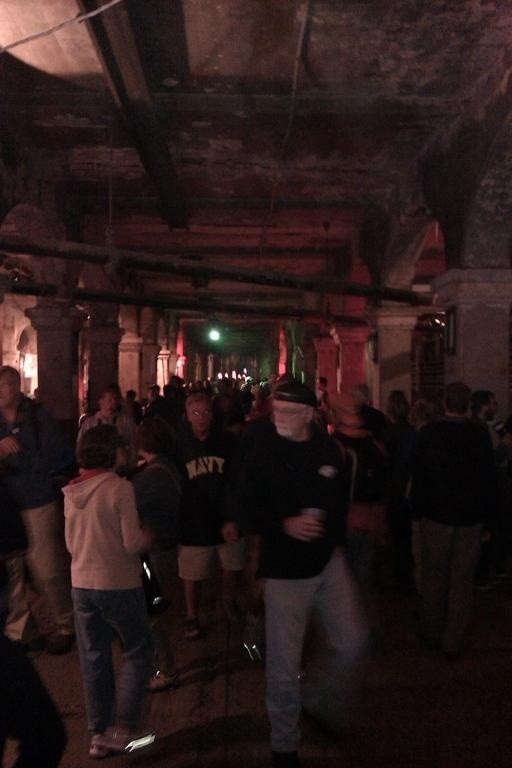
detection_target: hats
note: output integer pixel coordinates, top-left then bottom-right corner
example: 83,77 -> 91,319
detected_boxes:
335,398 -> 365,429
275,381 -> 317,405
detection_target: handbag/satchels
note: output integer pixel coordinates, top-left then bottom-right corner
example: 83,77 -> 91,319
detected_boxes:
346,502 -> 394,552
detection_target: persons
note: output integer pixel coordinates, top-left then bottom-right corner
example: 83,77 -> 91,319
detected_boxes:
1,365 -> 511,767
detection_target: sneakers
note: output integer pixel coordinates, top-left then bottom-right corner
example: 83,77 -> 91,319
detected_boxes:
145,669 -> 181,693
46,634 -> 75,654
272,751 -> 299,768
298,704 -> 337,748
183,613 -> 198,638
90,731 -> 143,758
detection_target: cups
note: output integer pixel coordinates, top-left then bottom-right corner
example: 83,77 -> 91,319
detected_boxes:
301,507 -> 325,537
